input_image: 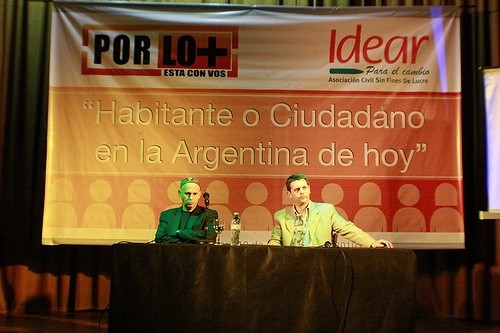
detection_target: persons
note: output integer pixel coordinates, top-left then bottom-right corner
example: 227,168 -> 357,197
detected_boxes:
154,176 -> 219,244
267,173 -> 395,249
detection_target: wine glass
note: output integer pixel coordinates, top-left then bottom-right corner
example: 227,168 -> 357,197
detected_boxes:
213,219 -> 225,246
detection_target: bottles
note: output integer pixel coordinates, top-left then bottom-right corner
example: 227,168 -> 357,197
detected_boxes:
230,212 -> 240,246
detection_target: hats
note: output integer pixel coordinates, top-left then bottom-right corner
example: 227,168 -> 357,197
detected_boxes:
180,176 -> 201,189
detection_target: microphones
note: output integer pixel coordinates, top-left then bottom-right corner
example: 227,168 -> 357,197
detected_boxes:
203,193 -> 209,205
